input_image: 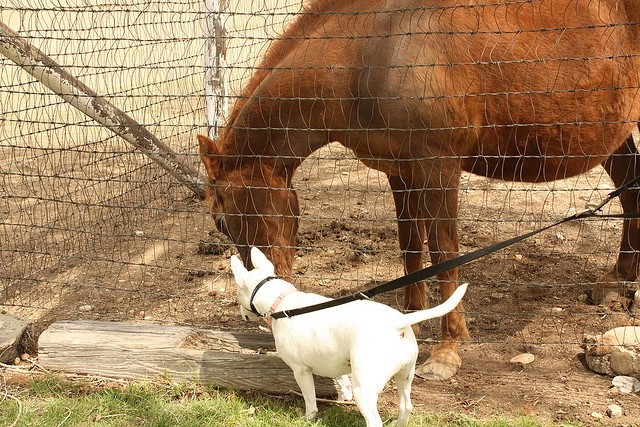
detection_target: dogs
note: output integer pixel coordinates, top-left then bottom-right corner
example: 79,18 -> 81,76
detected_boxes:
229,245 -> 470,427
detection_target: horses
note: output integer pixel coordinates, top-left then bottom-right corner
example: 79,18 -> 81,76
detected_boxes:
196,1 -> 640,382
